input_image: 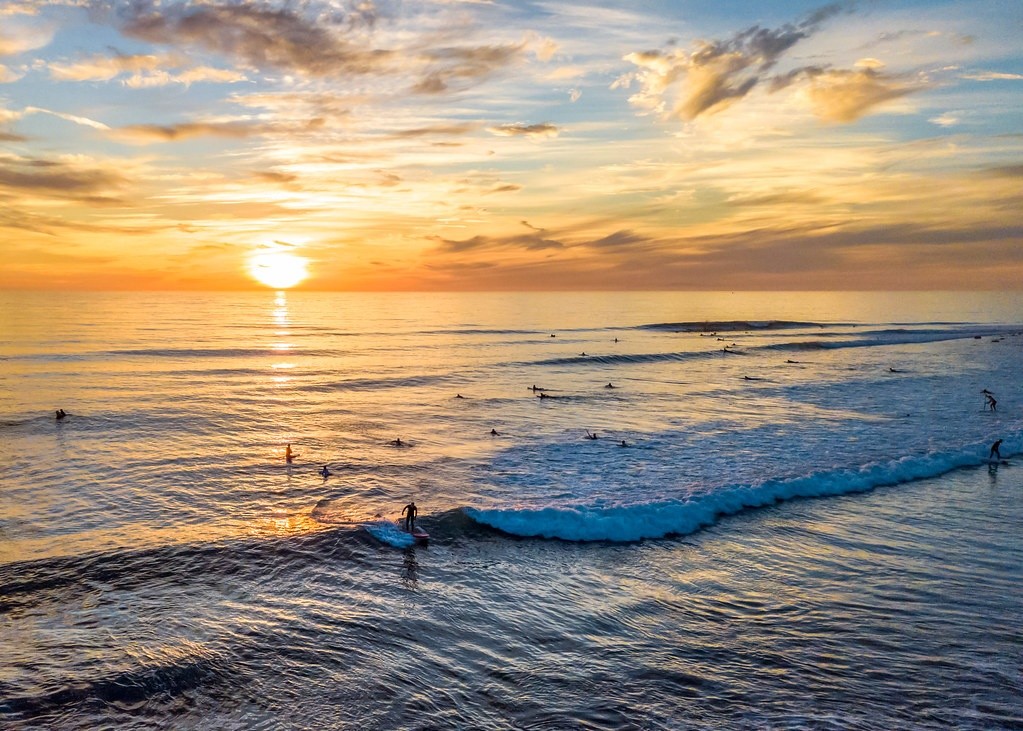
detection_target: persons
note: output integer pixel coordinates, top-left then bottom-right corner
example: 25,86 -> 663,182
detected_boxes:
54,330 -> 1023,535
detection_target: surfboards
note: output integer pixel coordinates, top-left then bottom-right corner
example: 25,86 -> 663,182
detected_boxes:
407,523 -> 429,538
